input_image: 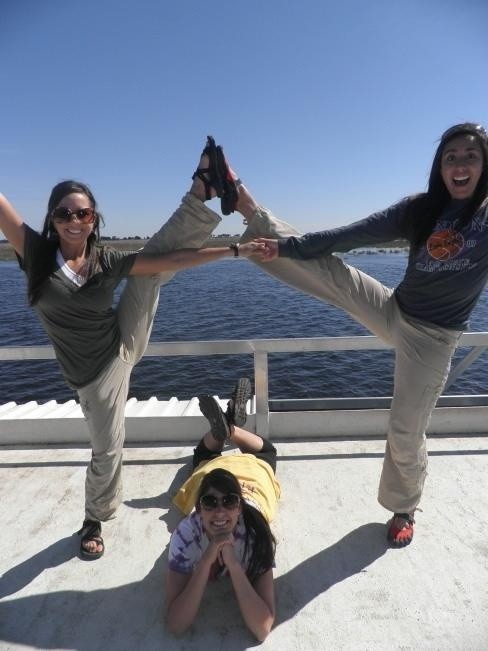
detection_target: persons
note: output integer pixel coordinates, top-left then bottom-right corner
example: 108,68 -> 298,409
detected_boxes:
0,136 -> 266,559
166,377 -> 281,642
216,123 -> 488,548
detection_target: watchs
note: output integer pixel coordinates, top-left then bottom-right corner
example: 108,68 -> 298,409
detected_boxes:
230,242 -> 240,257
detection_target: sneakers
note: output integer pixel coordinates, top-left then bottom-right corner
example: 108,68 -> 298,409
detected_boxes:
216,144 -> 243,216
227,377 -> 252,428
199,396 -> 231,441
387,512 -> 414,549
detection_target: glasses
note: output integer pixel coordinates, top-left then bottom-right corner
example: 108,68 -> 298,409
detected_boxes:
51,206 -> 97,226
200,494 -> 241,512
440,123 -> 487,141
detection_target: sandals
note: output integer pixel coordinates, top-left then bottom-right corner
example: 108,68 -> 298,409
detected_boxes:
191,135 -> 224,201
80,519 -> 104,559
